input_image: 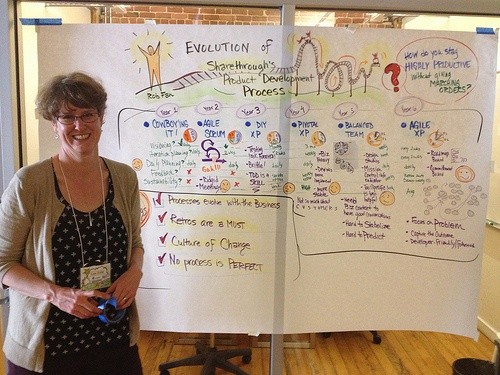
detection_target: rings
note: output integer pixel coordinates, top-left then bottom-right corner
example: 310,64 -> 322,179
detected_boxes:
123,297 -> 127,302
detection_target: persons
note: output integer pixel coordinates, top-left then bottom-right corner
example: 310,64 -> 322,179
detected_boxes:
1,72 -> 142,375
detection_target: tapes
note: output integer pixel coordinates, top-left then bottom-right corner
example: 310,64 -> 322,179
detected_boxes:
99,298 -> 125,323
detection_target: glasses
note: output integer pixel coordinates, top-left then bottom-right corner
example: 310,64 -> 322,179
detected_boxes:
55,111 -> 100,125
